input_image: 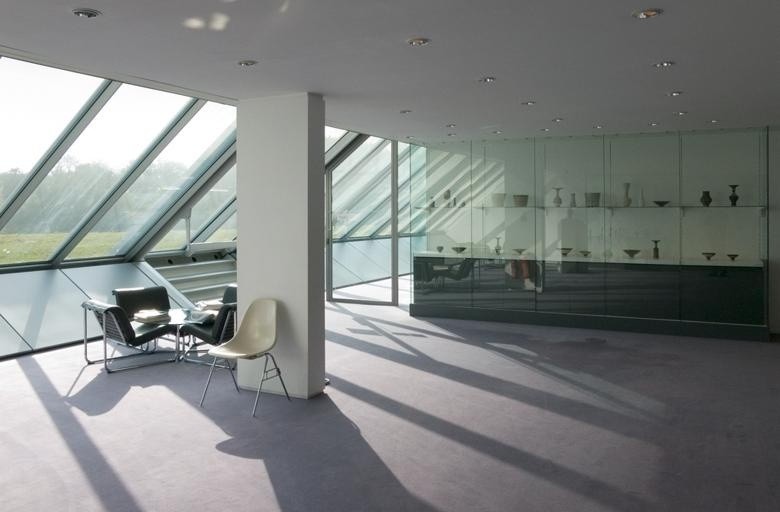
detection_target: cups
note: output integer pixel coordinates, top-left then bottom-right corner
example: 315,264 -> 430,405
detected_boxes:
182,308 -> 192,320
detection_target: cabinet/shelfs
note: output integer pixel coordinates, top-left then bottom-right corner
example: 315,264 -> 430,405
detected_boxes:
536,261 -> 605,328
678,264 -> 765,328
472,259 -> 536,323
414,258 -> 472,321
605,262 -> 679,328
409,124 -> 771,343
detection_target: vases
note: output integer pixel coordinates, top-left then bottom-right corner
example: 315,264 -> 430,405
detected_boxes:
551,187 -> 600,208
491,192 -> 528,207
494,237 -> 502,256
651,239 -> 661,260
621,182 -> 646,208
428,189 -> 465,209
700,184 -> 738,207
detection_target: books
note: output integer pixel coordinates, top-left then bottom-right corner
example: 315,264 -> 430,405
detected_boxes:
132,307 -> 171,323
195,300 -> 224,312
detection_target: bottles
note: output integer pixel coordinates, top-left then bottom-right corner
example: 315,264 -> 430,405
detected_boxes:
569,193 -> 576,208
700,191 -> 712,207
638,188 -> 646,208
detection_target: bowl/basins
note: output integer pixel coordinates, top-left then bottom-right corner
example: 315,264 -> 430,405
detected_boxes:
557,248 -> 573,256
653,201 -> 670,207
452,247 -> 465,253
513,249 -> 526,254
702,253 -> 739,261
624,250 -> 640,257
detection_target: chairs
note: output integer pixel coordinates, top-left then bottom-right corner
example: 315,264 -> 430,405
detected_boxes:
80,285 -> 291,418
